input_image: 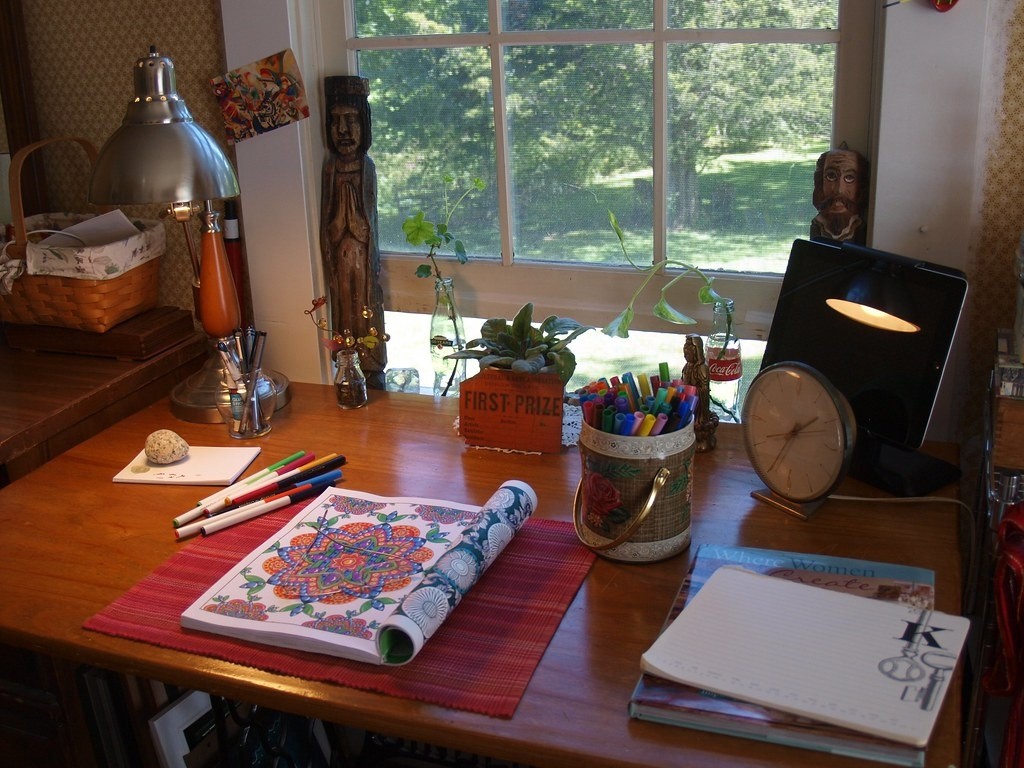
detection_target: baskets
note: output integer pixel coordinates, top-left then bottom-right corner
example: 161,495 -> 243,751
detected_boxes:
0,137 -> 167,333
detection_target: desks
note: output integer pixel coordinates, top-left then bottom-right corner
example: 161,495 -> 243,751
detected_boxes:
0,382 -> 963,768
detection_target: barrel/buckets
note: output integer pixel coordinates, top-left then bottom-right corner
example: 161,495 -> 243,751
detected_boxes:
572,414 -> 697,563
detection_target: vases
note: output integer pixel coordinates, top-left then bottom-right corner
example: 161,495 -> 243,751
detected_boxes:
333,350 -> 367,410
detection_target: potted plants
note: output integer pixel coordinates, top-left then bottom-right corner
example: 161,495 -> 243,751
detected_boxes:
400,175 -> 490,401
442,302 -> 598,384
597,205 -> 742,422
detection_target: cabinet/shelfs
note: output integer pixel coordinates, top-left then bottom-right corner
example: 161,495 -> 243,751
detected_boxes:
0,333 -> 206,491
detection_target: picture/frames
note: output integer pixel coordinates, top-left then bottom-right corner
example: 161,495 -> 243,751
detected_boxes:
147,689 -> 331,768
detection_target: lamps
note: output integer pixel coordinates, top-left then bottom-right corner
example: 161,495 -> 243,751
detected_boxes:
88,43 -> 293,425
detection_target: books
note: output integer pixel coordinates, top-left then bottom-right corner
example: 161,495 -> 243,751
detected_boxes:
627,544 -> 935,768
179,479 -> 538,666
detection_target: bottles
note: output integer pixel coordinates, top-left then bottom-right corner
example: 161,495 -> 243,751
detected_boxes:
214,365 -> 277,439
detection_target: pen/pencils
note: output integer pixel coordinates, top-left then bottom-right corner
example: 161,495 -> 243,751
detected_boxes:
171,450 -> 347,540
217,325 -> 268,435
579,362 -> 698,438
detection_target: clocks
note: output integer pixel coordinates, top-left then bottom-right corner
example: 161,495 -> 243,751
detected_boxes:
739,360 -> 858,521
